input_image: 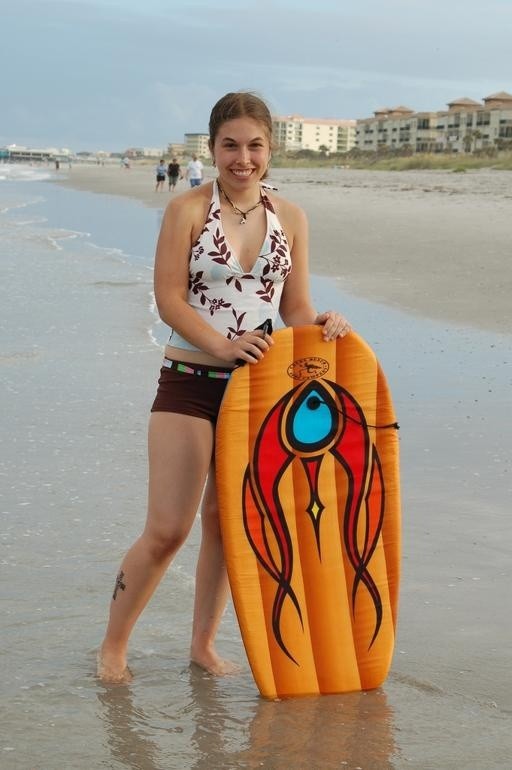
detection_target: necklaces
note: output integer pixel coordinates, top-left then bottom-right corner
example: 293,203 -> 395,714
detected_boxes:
217,179 -> 264,227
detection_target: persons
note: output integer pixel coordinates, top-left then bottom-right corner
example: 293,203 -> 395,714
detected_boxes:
95,663 -> 238,770
93,90 -> 353,680
167,158 -> 183,192
155,159 -> 167,192
185,155 -> 205,188
